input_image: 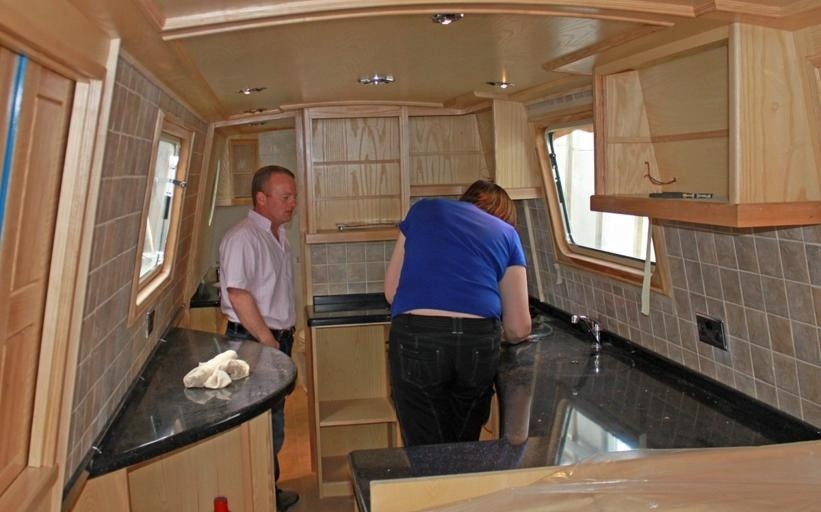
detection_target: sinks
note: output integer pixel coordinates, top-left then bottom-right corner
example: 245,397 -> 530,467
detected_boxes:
524,319 -> 553,340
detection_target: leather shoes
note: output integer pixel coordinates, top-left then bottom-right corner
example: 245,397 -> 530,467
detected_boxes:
275,487 -> 299,510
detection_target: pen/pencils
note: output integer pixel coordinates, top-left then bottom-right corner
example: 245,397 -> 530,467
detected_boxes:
649,192 -> 713,199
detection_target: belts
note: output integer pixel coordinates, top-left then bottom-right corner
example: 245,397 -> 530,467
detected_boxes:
226,320 -> 290,341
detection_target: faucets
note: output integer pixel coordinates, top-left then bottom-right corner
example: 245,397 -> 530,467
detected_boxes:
571,313 -> 601,344
571,352 -> 599,396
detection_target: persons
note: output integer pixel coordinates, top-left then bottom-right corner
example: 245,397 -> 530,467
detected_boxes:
405,346 -> 543,483
217,163 -> 301,512
382,178 -> 535,448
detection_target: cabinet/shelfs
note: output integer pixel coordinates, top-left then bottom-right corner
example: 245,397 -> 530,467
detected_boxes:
87,409 -> 298,512
307,304 -> 416,500
587,23 -> 820,229
211,96 -> 546,243
